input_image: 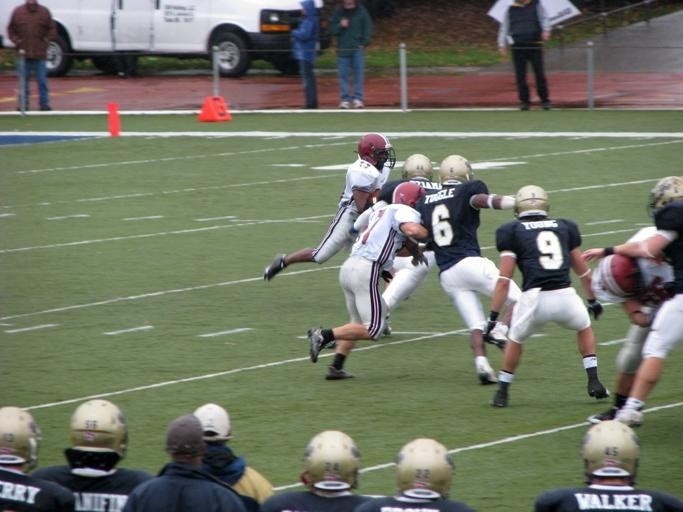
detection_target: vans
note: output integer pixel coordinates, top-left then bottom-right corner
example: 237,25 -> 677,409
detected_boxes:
0,1 -> 327,79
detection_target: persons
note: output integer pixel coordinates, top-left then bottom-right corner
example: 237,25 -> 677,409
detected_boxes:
0,397 -> 477,512
330,1 -> 373,109
263,135 -> 522,384
6,1 -> 57,111
484,185 -> 611,406
496,1 -> 552,110
582,176 -> 683,431
535,421 -> 682,511
291,1 -> 319,108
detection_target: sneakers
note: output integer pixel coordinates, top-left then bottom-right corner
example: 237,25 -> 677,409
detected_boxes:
265,253 -> 285,281
588,382 -> 611,400
614,408 -> 646,426
591,402 -> 625,423
326,367 -> 354,380
309,328 -> 326,364
339,99 -> 363,111
491,389 -> 508,408
383,328 -> 392,336
476,361 -> 496,384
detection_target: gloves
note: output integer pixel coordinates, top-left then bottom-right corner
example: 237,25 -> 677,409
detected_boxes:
483,330 -> 495,343
587,300 -> 602,320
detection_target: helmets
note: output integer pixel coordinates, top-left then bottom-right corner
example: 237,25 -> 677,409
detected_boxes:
579,420 -> 640,476
593,253 -> 642,301
647,176 -> 683,217
396,438 -> 453,498
70,397 -> 126,458
304,429 -> 358,488
357,132 -> 552,219
1,400 -> 40,465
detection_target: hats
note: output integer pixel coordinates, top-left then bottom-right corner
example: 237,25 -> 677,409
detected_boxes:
193,403 -> 232,441
165,418 -> 204,451
